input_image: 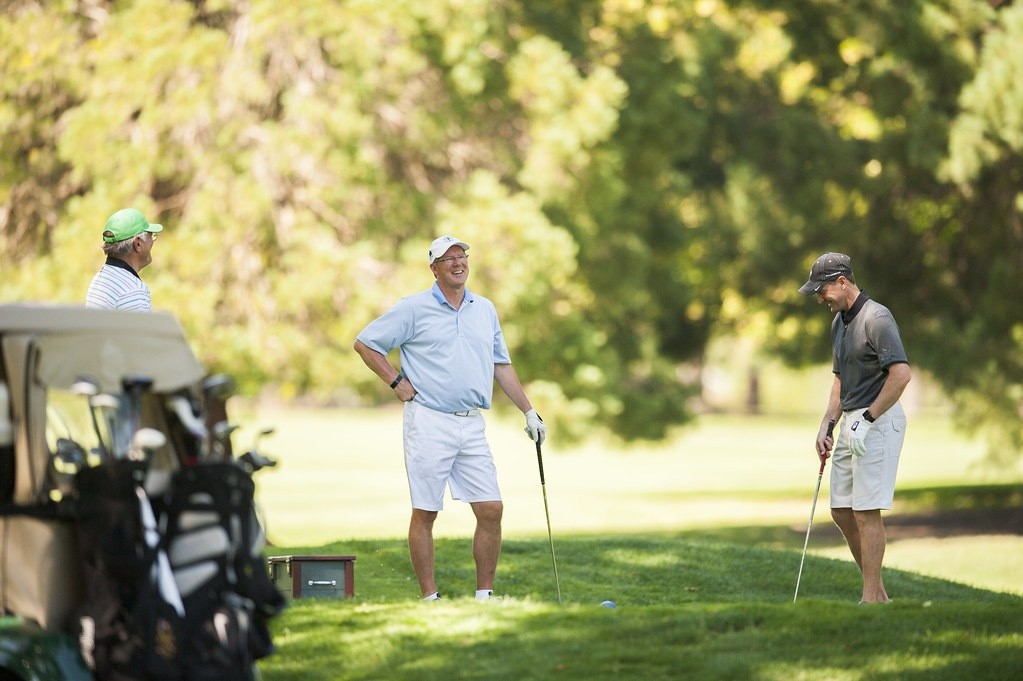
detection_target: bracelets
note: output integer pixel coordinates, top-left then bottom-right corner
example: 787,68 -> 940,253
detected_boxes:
391,375 -> 402,389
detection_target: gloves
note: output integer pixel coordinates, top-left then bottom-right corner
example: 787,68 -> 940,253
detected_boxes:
525,409 -> 546,445
848,410 -> 876,457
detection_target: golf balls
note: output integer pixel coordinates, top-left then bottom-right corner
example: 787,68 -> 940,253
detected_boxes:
600,600 -> 616,609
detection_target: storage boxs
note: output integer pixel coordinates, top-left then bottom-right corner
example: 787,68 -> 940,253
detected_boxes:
268,554 -> 355,601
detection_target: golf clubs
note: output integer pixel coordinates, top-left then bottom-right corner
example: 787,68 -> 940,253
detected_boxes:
534,429 -> 564,607
43,368 -> 280,473
793,418 -> 836,604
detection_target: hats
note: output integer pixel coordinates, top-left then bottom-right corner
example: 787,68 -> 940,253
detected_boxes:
798,251 -> 853,295
103,208 -> 164,243
428,235 -> 470,265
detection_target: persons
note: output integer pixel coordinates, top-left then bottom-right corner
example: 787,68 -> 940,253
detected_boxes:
355,235 -> 546,601
85,209 -> 164,313
799,251 -> 912,606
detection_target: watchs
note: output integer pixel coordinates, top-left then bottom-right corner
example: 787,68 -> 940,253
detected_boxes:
863,411 -> 875,423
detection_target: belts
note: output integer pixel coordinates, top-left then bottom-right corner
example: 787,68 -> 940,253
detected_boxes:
455,409 -> 482,416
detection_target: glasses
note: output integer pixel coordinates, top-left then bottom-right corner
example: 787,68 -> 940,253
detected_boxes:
139,233 -> 158,242
434,253 -> 470,266
